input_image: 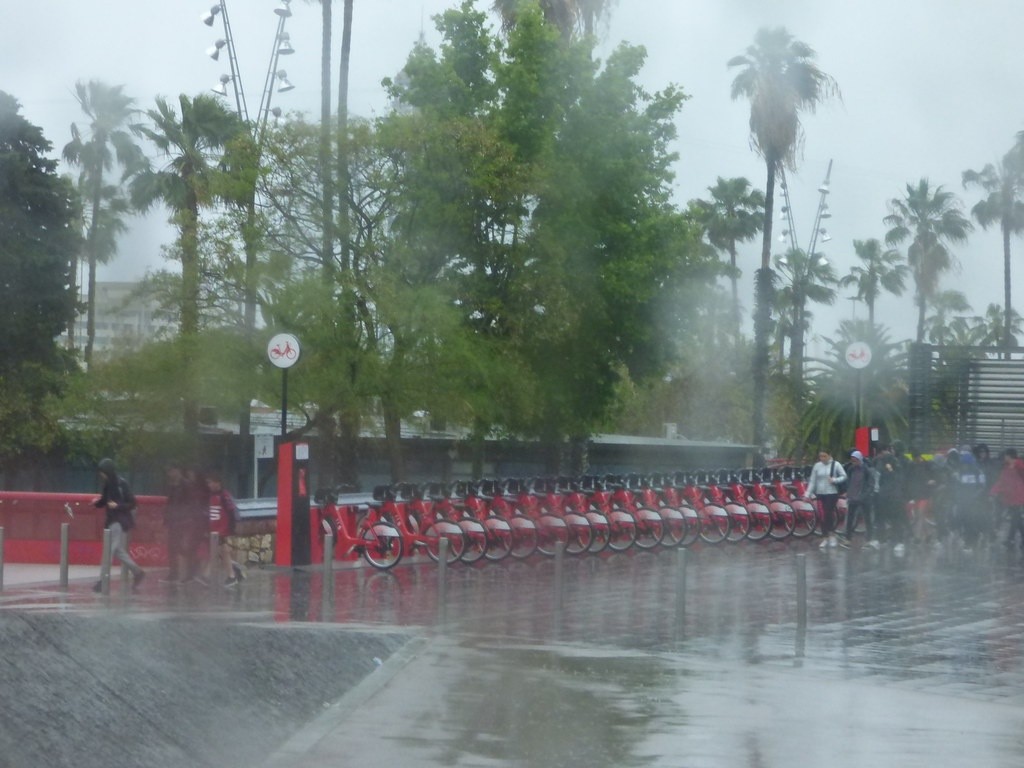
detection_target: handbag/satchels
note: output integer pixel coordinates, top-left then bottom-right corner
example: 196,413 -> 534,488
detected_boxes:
831,461 -> 846,494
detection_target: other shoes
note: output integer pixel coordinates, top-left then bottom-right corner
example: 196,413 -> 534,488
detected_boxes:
94,581 -> 101,592
132,571 -> 145,590
157,567 -> 239,589
818,529 -> 906,553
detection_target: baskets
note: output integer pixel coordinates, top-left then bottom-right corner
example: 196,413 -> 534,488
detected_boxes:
313,463 -> 812,505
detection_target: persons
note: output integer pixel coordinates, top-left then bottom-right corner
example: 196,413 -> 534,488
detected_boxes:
803,440 -> 1024,551
88,459 -> 146,594
157,468 -> 246,588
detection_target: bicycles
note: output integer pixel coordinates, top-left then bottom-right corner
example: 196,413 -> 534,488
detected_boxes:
318,467 -> 869,571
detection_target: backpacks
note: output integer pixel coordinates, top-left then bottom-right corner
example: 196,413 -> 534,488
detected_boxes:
117,478 -> 137,522
